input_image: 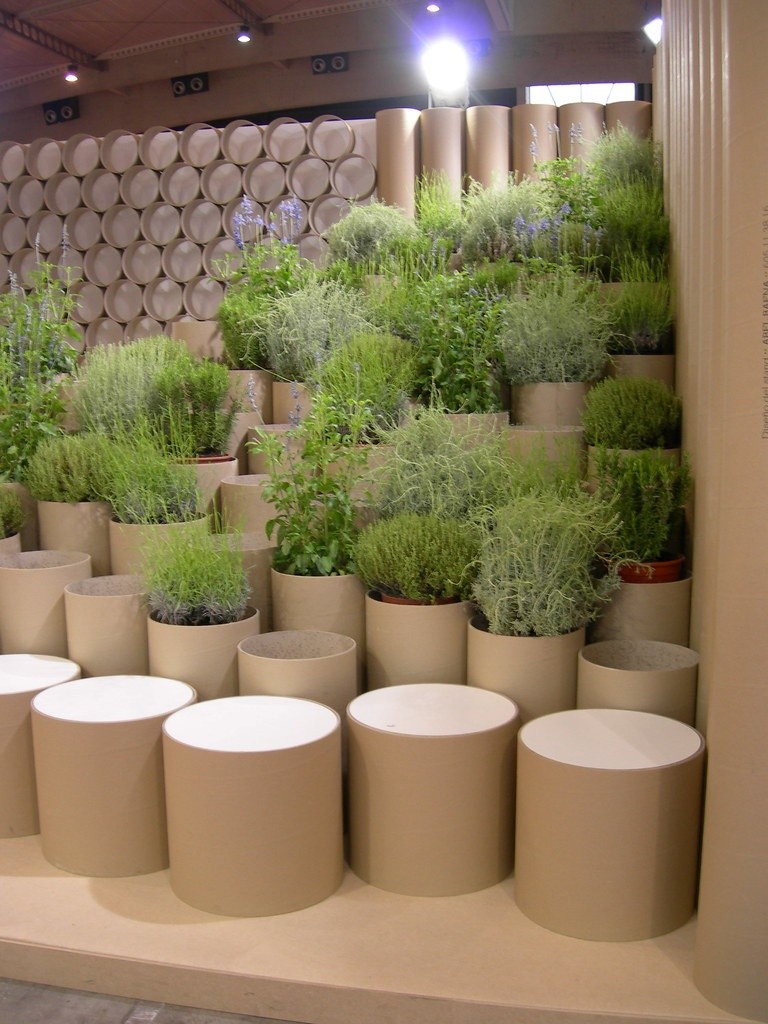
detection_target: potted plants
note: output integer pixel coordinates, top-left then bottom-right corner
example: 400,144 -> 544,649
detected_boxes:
0,117 -> 699,722
